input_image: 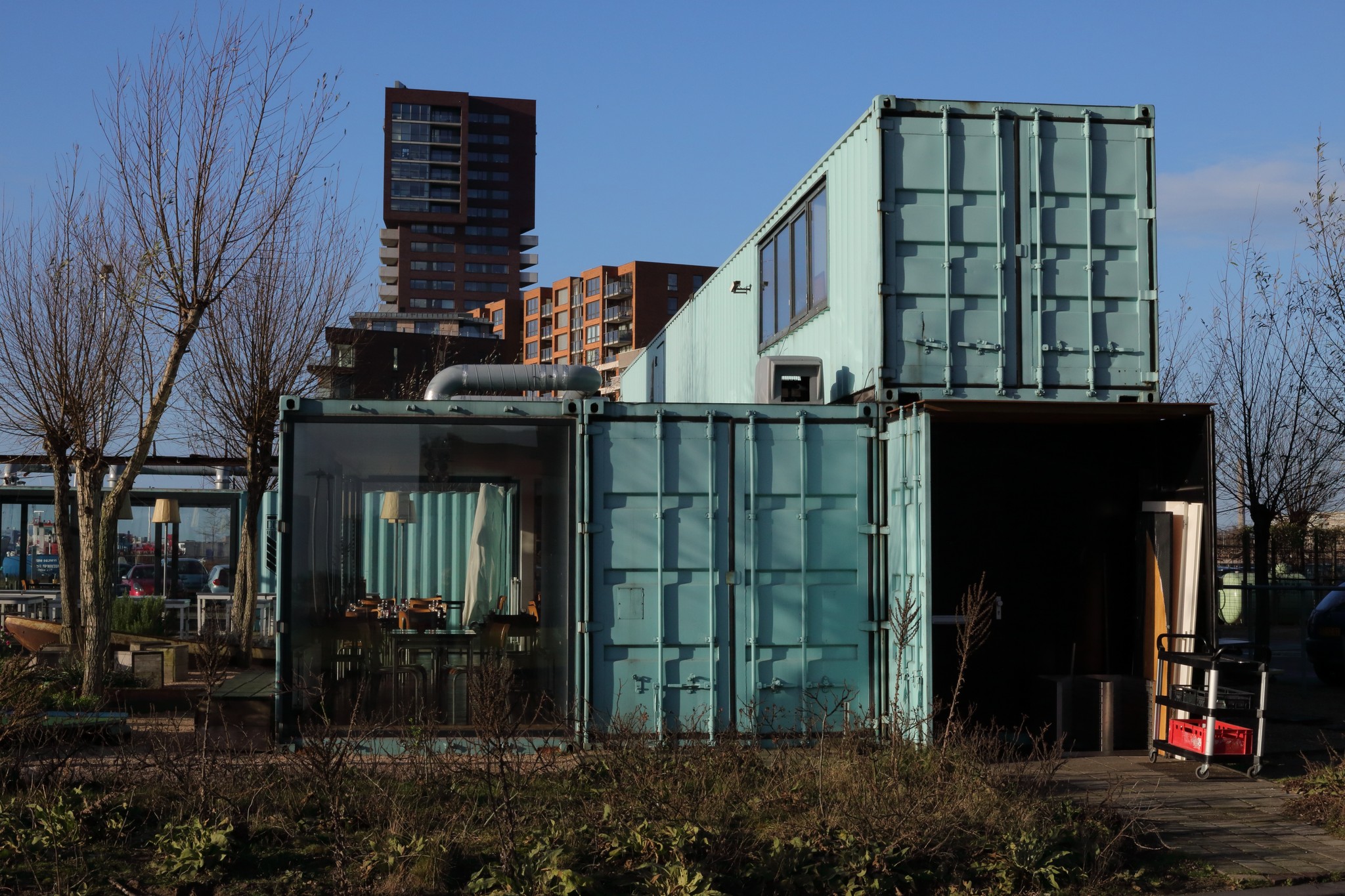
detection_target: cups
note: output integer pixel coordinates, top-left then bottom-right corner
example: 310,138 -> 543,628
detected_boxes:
381,600 -> 387,608
432,600 -> 438,608
520,608 -> 526,616
382,610 -> 389,618
52,579 -> 57,584
402,603 -> 408,612
350,603 -> 356,612
482,614 -> 489,623
489,608 -> 495,615
428,604 -> 434,612
399,606 -> 402,611
405,600 -> 410,607
434,610 -> 441,618
390,606 -> 397,618
440,605 -> 446,613
358,600 -> 363,607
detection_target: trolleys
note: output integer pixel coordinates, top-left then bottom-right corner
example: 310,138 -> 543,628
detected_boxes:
1149,632 -> 1272,780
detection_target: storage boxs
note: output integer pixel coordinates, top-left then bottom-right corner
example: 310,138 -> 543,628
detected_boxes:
1165,681 -> 1257,711
112,643 -> 189,688
1169,716 -> 1257,757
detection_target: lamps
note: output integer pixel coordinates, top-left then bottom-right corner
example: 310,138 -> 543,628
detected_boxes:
379,490 -> 416,526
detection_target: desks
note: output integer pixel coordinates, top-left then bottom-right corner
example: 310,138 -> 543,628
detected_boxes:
196,592 -> 276,638
389,600 -> 477,728
0,582 -> 81,639
116,595 -> 191,640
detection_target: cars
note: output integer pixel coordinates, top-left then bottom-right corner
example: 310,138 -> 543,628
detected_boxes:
117,563 -> 133,585
201,564 -> 229,593
121,564 -> 189,600
1305,581 -> 1345,686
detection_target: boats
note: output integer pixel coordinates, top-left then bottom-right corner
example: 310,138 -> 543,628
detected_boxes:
3,614 -> 277,672
1,510 -> 61,585
159,557 -> 209,604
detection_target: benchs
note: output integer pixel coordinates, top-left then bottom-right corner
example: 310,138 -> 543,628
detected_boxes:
194,670 -> 278,753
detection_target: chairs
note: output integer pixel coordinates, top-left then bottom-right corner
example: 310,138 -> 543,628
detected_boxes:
22,579 -> 49,620
336,595 -> 542,729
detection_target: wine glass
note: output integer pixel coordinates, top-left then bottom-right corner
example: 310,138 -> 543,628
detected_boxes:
378,604 -> 383,618
